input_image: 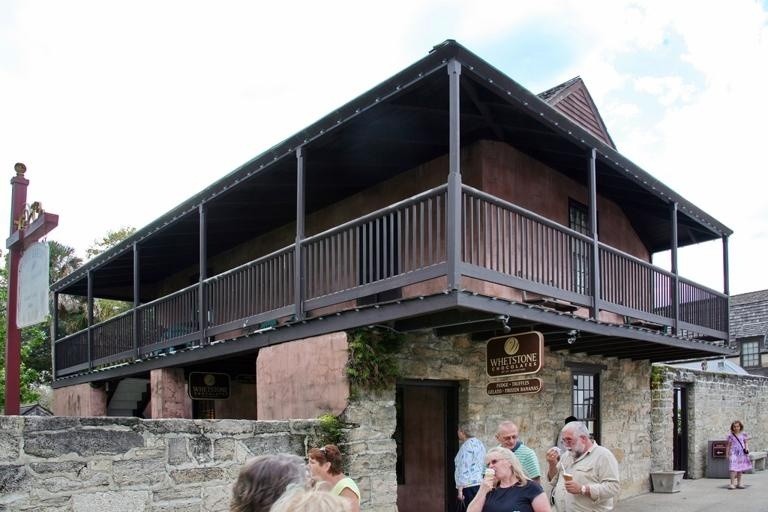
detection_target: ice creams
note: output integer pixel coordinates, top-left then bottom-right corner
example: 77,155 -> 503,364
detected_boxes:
484,469 -> 496,483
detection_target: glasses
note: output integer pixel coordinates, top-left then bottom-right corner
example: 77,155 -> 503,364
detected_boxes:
319,447 -> 329,462
486,459 -> 499,467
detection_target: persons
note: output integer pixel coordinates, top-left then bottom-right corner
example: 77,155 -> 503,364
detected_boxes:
266,473 -> 352,512
304,442 -> 363,512
724,419 -> 754,490
226,451 -> 311,512
466,444 -> 552,511
493,418 -> 543,486
451,419 -> 486,509
546,418 -> 624,511
555,414 -> 578,460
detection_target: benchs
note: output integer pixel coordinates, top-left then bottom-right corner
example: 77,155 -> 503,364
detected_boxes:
744,452 -> 767,474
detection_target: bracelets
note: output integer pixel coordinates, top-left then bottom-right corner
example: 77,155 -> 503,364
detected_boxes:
580,484 -> 587,495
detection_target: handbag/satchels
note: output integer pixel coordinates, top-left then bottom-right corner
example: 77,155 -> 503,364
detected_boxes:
743,449 -> 748,454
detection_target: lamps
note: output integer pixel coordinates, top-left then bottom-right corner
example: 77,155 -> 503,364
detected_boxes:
567,330 -> 577,345
502,319 -> 511,333
